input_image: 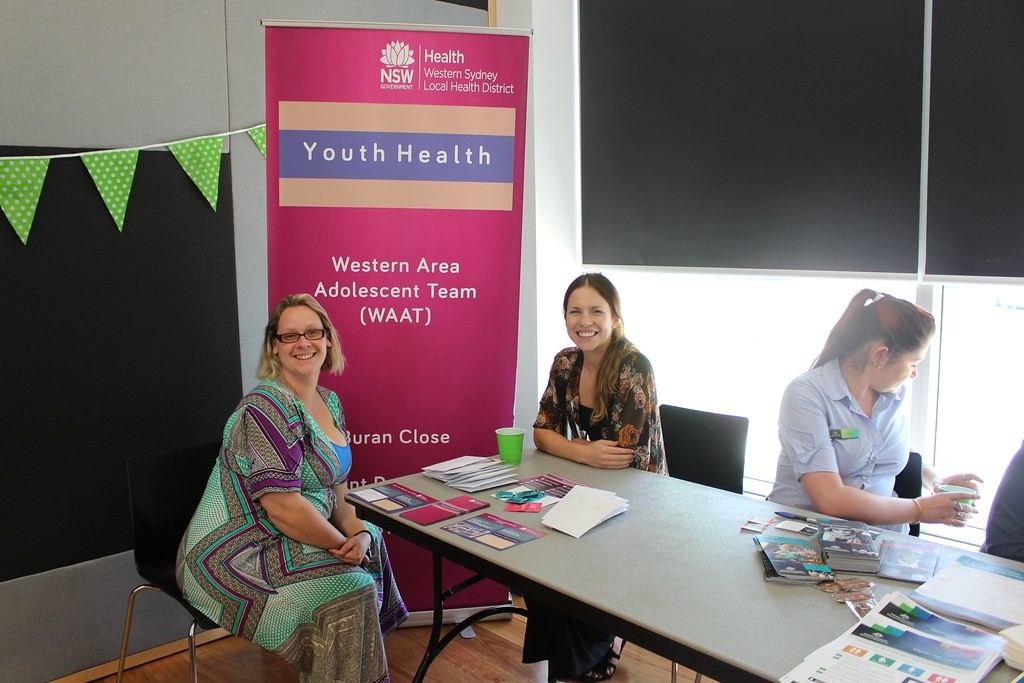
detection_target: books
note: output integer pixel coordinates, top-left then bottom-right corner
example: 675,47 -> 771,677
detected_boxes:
878,540 -> 945,583
753,534 -> 833,585
909,554 -> 1024,631
815,516 -> 880,575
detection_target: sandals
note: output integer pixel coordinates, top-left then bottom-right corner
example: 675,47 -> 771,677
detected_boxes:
584,640 -> 626,681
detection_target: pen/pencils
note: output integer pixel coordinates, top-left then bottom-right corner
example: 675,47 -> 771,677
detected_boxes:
776,512 -> 818,524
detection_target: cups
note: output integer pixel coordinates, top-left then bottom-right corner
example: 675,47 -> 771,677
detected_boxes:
495,428 -> 526,465
940,485 -> 976,527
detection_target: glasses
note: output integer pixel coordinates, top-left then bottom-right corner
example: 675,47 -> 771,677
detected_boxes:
274,329 -> 326,343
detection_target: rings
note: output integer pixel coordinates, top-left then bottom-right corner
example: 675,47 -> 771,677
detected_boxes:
962,474 -> 964,479
951,520 -> 954,526
956,512 -> 960,518
957,503 -> 962,511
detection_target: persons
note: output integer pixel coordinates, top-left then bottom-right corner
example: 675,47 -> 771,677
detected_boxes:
980,440 -> 1024,564
175,293 -> 410,683
824,527 -> 875,554
519,273 -> 668,683
764,289 -> 984,535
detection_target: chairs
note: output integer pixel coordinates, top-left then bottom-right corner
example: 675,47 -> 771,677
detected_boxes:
118,439 -> 221,683
659,403 -> 748,683
894,452 -> 922,539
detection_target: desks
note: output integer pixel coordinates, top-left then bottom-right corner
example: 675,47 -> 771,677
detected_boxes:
341,446 -> 1024,683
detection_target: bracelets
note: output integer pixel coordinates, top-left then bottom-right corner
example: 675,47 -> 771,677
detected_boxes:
910,500 -> 922,525
354,530 -> 374,557
